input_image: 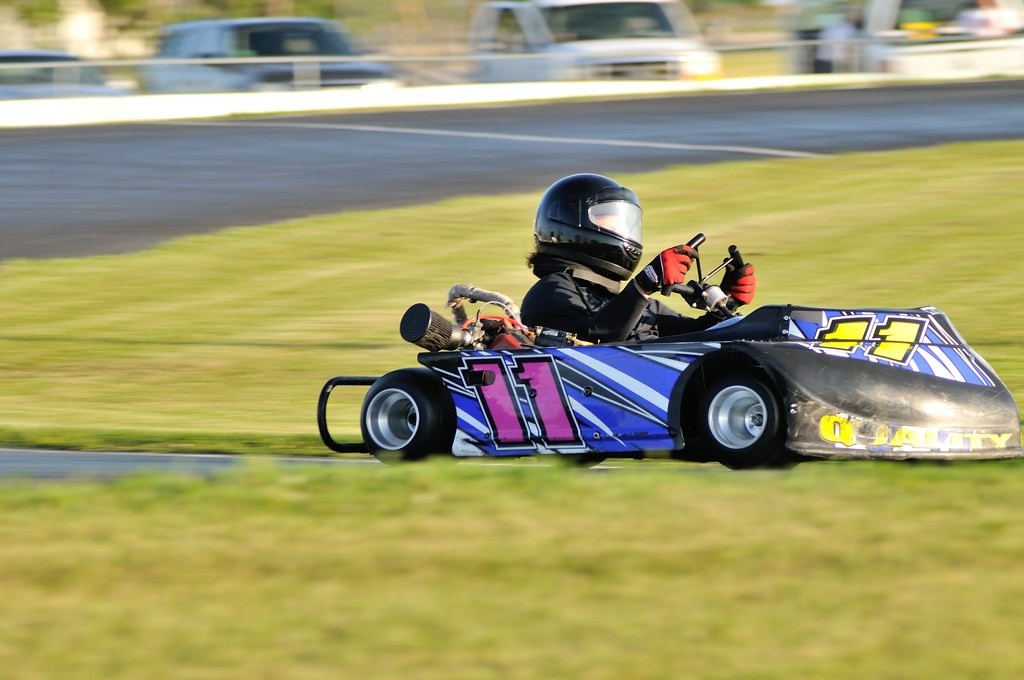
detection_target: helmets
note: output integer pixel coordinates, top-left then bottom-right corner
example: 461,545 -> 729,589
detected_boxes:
534,172 -> 643,280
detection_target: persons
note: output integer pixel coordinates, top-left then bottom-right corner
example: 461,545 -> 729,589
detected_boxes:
814,7 -> 859,73
954,0 -> 1024,34
520,174 -> 754,344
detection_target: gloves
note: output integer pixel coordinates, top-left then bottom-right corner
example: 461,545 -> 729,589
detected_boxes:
635,245 -> 699,295
706,258 -> 757,320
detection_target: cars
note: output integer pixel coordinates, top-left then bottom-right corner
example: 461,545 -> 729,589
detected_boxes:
1,51 -> 140,97
136,14 -> 406,95
470,1 -> 720,80
